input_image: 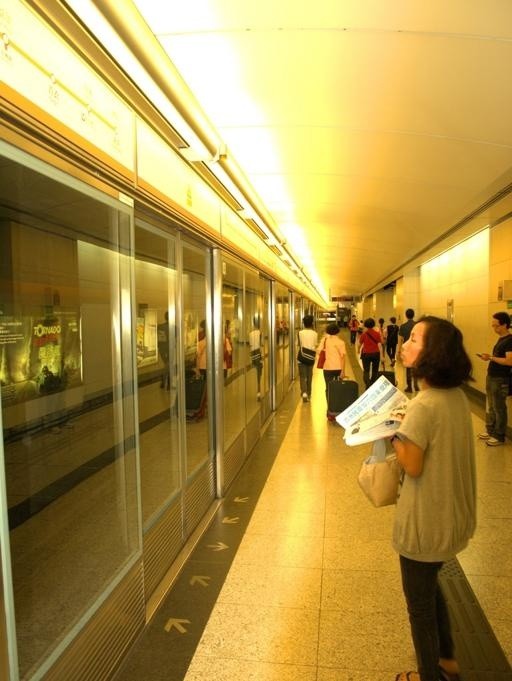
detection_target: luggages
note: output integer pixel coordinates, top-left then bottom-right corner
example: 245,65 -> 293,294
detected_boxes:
327,375 -> 359,417
372,361 -> 396,387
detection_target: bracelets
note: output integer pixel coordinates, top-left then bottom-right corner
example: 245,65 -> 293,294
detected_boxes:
389,436 -> 400,446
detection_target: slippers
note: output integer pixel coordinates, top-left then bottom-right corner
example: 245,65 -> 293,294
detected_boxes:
394,664 -> 458,681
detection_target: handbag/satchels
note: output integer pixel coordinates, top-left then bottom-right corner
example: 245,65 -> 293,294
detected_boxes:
357,453 -> 401,509
317,350 -> 326,368
296,347 -> 316,366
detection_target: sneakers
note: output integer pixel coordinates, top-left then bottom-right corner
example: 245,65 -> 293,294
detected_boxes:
478,432 -> 491,439
486,437 -> 504,446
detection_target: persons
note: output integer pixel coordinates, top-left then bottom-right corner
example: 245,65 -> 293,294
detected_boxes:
275,317 -> 281,346
249,315 -> 265,402
223,319 -> 235,380
383,313 -> 481,679
192,316 -> 208,420
294,306 -> 512,446
282,313 -> 287,347
157,309 -> 172,395
